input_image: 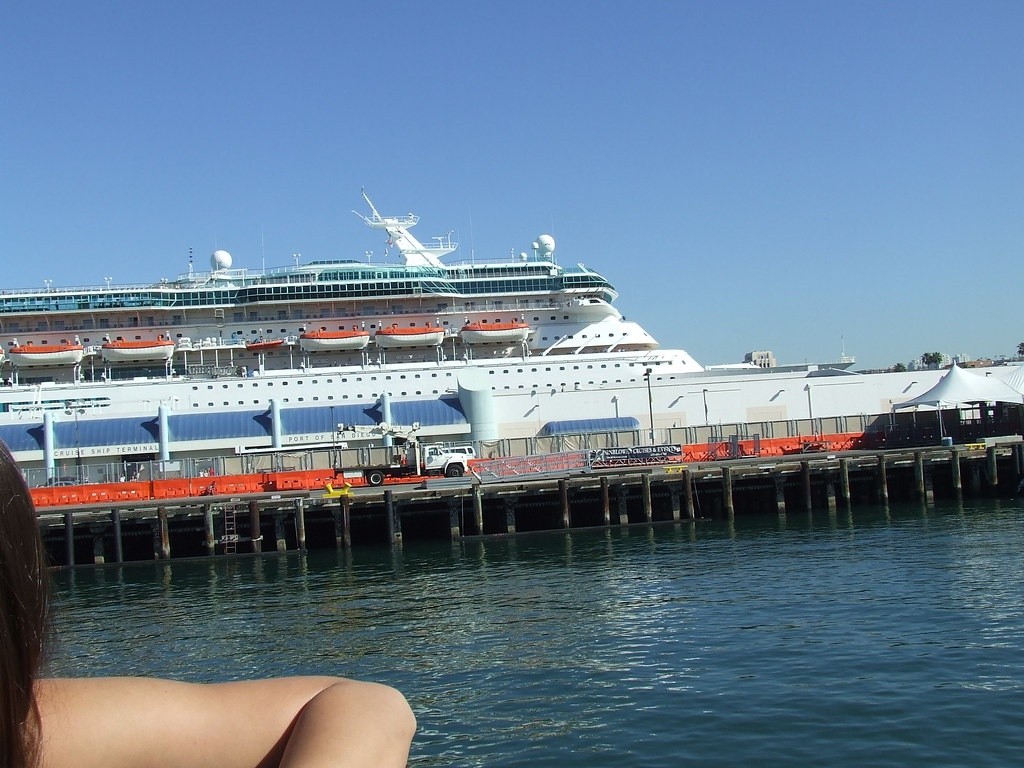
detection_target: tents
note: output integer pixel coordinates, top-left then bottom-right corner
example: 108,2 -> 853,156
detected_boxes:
893,358 -> 1024,444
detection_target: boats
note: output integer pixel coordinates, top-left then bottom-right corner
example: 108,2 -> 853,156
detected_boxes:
100,330 -> 175,362
245,338 -> 284,350
461,313 -> 529,344
8,335 -> 84,366
299,321 -> 371,351
375,317 -> 445,348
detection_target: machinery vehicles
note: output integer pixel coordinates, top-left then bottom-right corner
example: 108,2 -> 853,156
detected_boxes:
328,421 -> 470,487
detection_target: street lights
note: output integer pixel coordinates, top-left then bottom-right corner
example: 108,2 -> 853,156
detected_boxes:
646,367 -> 655,445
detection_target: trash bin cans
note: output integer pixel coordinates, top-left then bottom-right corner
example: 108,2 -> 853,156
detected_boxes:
941,436 -> 953,447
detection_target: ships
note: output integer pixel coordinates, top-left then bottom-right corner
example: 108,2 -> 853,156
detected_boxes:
0,181 -> 1024,488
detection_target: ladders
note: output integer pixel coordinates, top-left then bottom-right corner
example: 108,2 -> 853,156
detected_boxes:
187,363 -> 215,375
224,503 -> 237,556
211,366 -> 247,378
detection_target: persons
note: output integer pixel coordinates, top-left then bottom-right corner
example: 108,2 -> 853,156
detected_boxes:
1,440 -> 417,768
199,466 -> 217,496
117,470 -> 137,482
491,451 -> 496,460
